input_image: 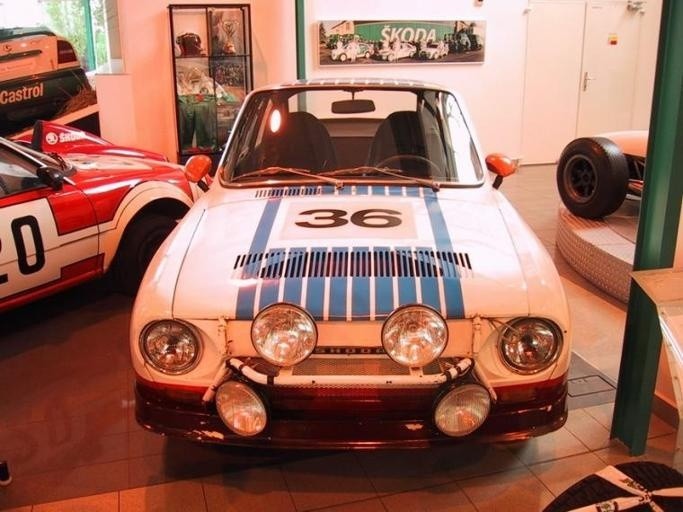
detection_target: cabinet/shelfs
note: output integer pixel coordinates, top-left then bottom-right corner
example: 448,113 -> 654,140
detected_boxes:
164,3 -> 256,167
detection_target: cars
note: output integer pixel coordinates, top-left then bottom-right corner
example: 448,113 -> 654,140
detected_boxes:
557,129 -> 650,221
0,25 -> 82,137
0,120 -> 215,314
129,77 -> 573,452
330,40 -> 449,62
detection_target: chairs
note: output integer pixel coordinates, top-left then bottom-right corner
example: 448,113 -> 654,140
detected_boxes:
362,108 -> 453,182
257,109 -> 338,177
